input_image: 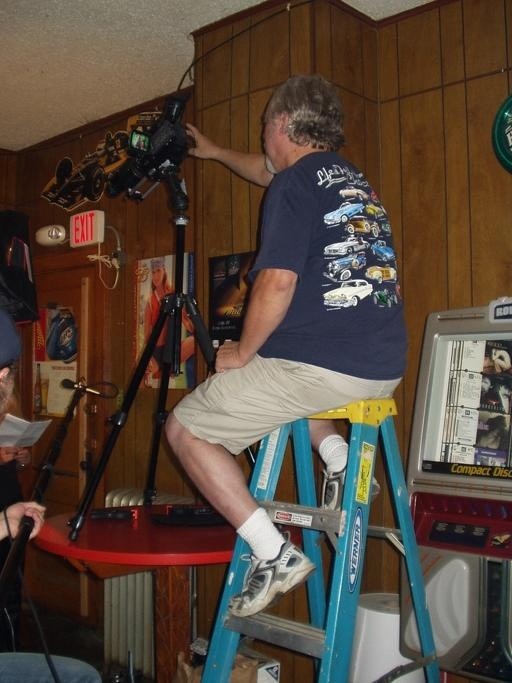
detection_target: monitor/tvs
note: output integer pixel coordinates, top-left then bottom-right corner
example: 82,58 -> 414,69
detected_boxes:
406,295 -> 512,502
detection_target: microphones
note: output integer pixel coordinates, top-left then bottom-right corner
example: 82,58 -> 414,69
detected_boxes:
60,379 -> 103,399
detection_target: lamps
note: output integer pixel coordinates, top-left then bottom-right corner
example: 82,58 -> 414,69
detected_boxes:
34,224 -> 66,247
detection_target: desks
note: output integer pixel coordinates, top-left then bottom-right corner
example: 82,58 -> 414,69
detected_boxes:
34,502 -> 302,683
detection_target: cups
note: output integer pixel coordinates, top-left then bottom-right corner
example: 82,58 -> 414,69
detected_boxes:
40,374 -> 49,416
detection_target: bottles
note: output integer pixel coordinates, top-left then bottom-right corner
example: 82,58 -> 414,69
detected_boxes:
33,362 -> 43,415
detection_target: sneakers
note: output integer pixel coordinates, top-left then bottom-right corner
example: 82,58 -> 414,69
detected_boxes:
321,464 -> 381,512
227,541 -> 318,618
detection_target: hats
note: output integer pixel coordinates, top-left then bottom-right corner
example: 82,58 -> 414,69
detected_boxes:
150,256 -> 165,267
0,308 -> 22,369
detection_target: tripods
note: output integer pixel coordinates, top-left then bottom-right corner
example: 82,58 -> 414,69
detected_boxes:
68,177 -> 256,544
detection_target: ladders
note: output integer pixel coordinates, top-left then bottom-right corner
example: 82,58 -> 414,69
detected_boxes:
201,399 -> 439,683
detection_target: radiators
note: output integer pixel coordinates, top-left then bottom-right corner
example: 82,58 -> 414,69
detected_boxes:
103,487 -> 198,682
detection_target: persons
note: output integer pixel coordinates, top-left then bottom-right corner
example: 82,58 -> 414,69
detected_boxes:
0,310 -> 100,683
164,75 -> 406,617
145,260 -> 195,379
